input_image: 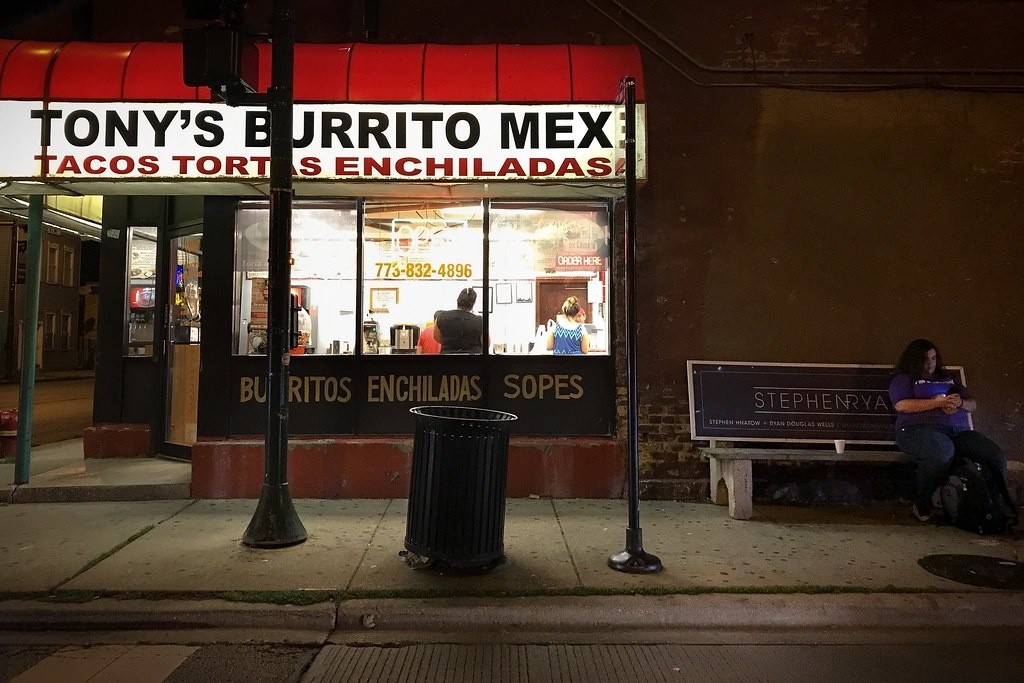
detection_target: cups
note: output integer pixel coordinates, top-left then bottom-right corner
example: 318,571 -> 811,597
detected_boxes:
835,441 -> 846,453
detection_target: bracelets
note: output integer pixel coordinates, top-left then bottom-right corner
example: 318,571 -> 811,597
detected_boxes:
957,398 -> 964,409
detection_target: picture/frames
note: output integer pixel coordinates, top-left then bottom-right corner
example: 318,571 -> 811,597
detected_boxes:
496,283 -> 512,304
370,288 -> 399,312
516,281 -> 532,303
472,286 -> 493,313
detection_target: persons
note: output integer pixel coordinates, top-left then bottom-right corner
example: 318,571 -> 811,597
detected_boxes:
887,336 -> 1024,536
433,287 -> 490,354
290,286 -> 313,345
546,295 -> 597,355
416,310 -> 446,355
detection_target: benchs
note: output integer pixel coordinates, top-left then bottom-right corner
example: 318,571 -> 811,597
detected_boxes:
687,359 -> 977,520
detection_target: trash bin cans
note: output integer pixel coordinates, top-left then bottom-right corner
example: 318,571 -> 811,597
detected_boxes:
405,406 -> 517,577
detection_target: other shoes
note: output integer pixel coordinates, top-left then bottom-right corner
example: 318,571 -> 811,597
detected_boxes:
911,504 -> 930,521
